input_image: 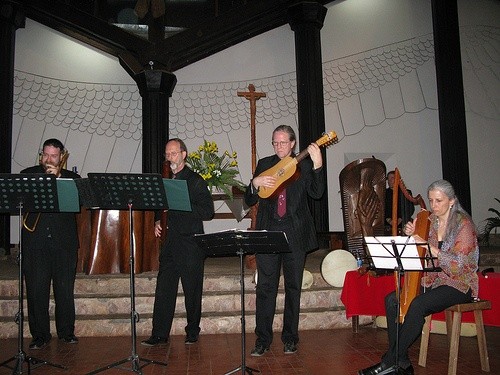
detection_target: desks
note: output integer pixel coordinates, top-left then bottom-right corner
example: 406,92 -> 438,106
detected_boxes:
340,271 -> 500,335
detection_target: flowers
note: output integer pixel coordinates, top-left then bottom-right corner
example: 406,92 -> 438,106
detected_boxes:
186,138 -> 248,201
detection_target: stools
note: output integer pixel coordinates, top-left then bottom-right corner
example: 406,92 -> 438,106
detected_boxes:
418,300 -> 492,375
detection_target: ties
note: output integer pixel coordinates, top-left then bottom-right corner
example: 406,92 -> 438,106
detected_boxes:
278,188 -> 286,217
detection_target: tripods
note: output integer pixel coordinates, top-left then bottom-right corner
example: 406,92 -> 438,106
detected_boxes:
82,173 -> 193,375
0,172 -> 81,375
194,229 -> 293,375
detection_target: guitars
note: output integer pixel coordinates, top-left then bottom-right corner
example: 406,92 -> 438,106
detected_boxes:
255,129 -> 339,201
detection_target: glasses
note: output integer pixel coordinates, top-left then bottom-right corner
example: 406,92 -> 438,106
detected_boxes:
166,152 -> 182,156
272,141 -> 292,146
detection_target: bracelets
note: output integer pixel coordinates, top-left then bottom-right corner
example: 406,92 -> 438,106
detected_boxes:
427,243 -> 432,249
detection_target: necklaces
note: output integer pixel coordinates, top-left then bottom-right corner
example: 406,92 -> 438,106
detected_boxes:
436,218 -> 446,230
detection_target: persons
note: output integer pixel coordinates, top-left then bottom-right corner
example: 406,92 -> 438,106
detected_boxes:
10,138 -> 82,350
358,180 -> 479,375
382,170 -> 415,236
141,138 -> 215,346
244,125 -> 326,356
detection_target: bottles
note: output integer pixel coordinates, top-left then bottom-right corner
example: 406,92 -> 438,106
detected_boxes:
356,249 -> 363,266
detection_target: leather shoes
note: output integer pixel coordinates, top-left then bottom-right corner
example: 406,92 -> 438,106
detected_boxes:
186,336 -> 198,345
28,336 -> 52,350
250,346 -> 270,356
283,343 -> 299,354
59,338 -> 80,344
141,337 -> 170,347
356,360 -> 400,375
401,365 -> 414,375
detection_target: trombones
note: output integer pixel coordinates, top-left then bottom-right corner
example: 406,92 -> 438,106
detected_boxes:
23,149 -> 70,232
